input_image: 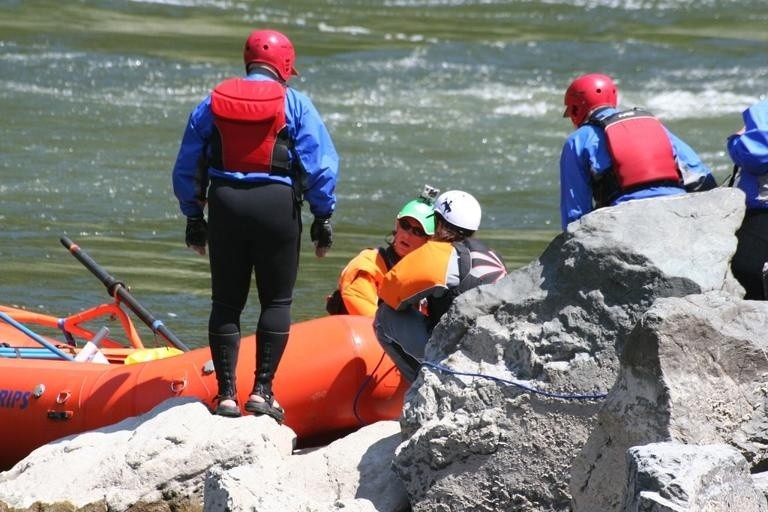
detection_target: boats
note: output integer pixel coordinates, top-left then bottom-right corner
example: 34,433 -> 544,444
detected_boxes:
1,300 -> 412,473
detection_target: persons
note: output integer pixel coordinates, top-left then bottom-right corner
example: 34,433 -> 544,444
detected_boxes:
371,188 -> 508,385
557,72 -> 718,233
724,93 -> 767,300
337,194 -> 435,316
171,28 -> 340,426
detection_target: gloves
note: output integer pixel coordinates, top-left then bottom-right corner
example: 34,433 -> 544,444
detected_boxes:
184,215 -> 208,249
310,217 -> 334,250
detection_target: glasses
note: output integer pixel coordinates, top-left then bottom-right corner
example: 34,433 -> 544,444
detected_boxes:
398,220 -> 427,237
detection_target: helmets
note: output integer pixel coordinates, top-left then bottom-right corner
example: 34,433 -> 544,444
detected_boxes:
242,28 -> 299,83
425,188 -> 484,232
559,71 -> 619,128
395,197 -> 436,237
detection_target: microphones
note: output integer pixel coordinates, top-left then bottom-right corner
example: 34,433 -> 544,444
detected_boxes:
384,229 -> 399,244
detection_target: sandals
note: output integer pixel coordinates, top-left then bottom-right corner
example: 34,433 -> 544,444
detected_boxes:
243,389 -> 288,422
212,391 -> 241,419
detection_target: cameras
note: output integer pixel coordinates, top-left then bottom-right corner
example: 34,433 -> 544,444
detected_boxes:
420,184 -> 440,202
